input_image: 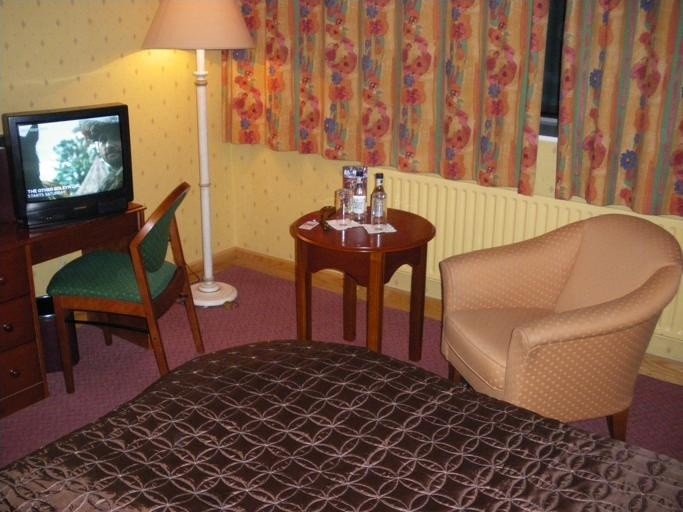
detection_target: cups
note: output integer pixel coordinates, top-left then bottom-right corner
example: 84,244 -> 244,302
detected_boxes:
371,192 -> 388,230
335,189 -> 352,225
336,226 -> 350,247
371,230 -> 385,249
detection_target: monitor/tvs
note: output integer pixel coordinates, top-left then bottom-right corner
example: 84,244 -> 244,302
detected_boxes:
0,102 -> 134,229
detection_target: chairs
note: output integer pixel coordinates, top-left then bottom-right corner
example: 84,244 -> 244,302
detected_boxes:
46,182 -> 206,394
438,215 -> 682,441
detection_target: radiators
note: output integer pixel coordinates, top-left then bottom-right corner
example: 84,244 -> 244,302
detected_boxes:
366,166 -> 683,362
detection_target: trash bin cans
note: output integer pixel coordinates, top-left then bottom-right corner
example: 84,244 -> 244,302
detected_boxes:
35,294 -> 80,373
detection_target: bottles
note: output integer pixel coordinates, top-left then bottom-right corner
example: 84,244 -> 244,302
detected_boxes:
353,169 -> 368,221
371,172 -> 386,198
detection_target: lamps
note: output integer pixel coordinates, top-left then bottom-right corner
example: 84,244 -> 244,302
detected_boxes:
140,0 -> 256,309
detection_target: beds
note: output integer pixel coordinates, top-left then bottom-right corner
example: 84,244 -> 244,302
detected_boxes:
0,337 -> 682,511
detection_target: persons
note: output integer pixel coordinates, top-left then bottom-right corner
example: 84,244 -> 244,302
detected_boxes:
82,117 -> 123,194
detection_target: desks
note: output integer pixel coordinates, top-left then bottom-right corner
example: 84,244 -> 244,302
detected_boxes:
0,201 -> 147,421
290,205 -> 436,371
